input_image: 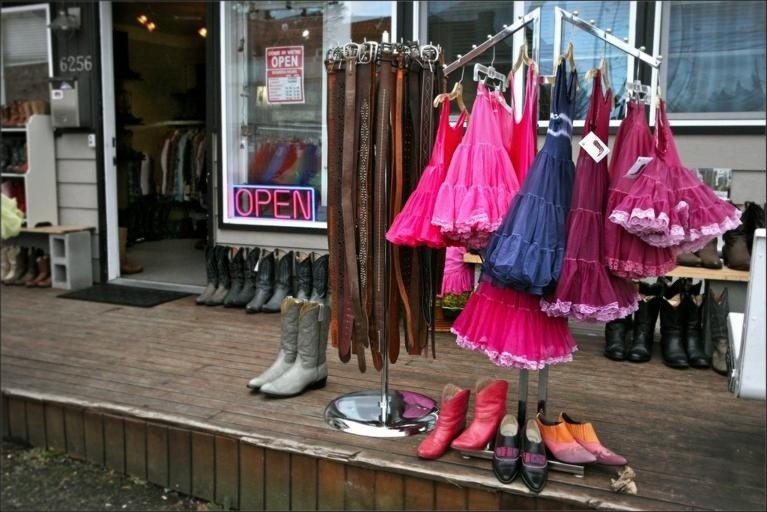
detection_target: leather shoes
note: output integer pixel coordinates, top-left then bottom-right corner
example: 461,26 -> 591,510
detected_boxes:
419,378 -> 628,493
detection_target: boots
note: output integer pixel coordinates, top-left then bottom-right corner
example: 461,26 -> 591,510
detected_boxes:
118,228 -> 142,274
0,244 -> 51,287
0,100 -> 45,126
0,141 -> 27,173
196,246 -> 329,396
604,201 -> 766,374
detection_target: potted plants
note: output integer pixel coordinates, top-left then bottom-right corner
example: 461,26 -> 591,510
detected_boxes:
439,291 -> 472,323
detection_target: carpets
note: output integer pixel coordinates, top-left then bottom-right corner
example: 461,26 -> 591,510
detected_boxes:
56,279 -> 196,309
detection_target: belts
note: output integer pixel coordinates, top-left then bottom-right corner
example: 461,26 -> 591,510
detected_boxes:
324,41 -> 444,373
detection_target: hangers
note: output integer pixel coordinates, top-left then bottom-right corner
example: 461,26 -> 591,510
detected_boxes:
432,13 -> 663,119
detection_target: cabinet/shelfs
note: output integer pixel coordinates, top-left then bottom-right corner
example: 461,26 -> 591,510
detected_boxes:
0,113 -> 59,229
